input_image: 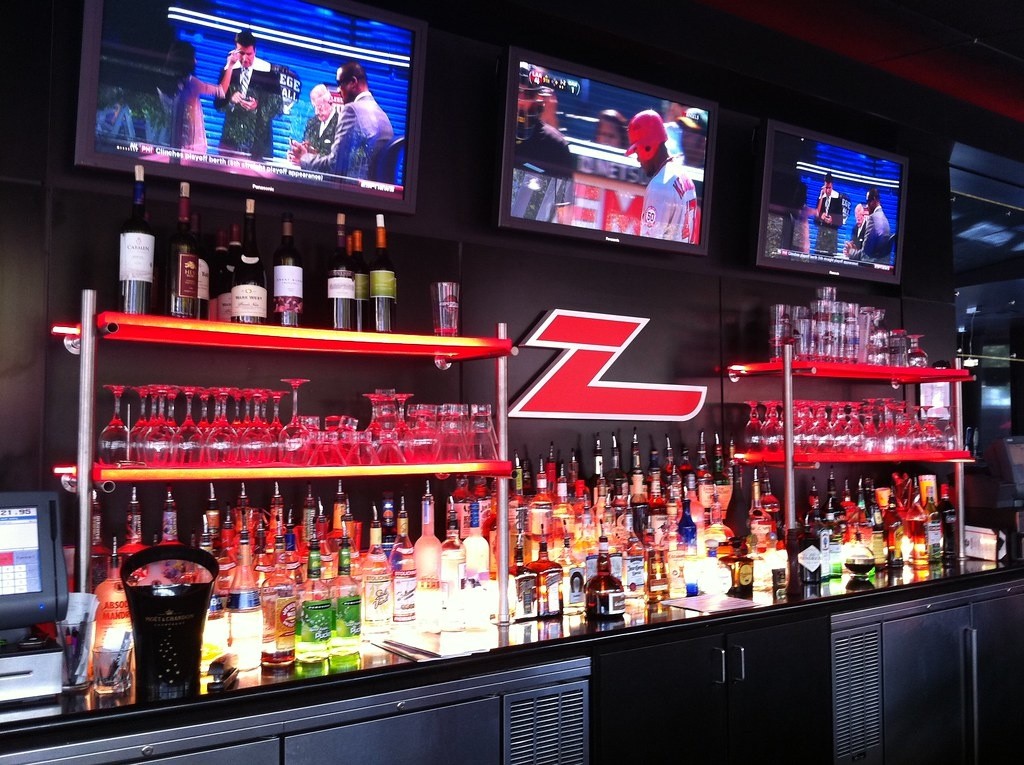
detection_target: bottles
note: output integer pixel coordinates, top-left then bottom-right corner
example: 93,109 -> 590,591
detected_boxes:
87,426 -> 956,680
117,164 -> 396,332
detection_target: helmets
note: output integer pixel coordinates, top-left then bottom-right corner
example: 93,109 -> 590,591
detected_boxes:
625,109 -> 668,162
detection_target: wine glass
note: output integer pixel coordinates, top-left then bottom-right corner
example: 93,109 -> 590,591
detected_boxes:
741,397 -> 956,452
906,334 -> 928,367
362,392 -> 413,445
97,375 -> 312,466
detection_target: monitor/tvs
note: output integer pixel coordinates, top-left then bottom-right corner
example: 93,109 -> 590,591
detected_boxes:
74,0 -> 428,214
0,491 -> 68,629
496,45 -> 718,256
756,118 -> 909,285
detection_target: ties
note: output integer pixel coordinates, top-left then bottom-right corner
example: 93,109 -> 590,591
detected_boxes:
241,67 -> 250,96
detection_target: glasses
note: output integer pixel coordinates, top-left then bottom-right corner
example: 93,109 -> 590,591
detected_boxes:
335,78 -> 348,87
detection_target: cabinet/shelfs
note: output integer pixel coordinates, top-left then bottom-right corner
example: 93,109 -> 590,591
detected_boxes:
713,343 -> 977,561
0,578 -> 1024,765
52,287 -> 518,628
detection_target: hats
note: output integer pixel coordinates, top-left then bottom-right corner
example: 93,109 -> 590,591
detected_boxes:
673,107 -> 709,136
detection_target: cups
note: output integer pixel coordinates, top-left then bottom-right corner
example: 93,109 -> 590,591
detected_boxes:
768,286 -> 909,365
91,646 -> 133,695
430,281 -> 460,336
55,620 -> 96,691
298,400 -> 498,463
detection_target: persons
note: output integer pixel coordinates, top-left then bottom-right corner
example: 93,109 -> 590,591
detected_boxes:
302,84 -> 342,160
521,86 -> 708,170
287,62 -> 394,184
791,182 -> 826,253
162,41 -> 241,155
843,188 -> 891,265
623,110 -> 697,245
814,172 -> 843,256
510,61 -> 576,227
213,31 -> 283,163
